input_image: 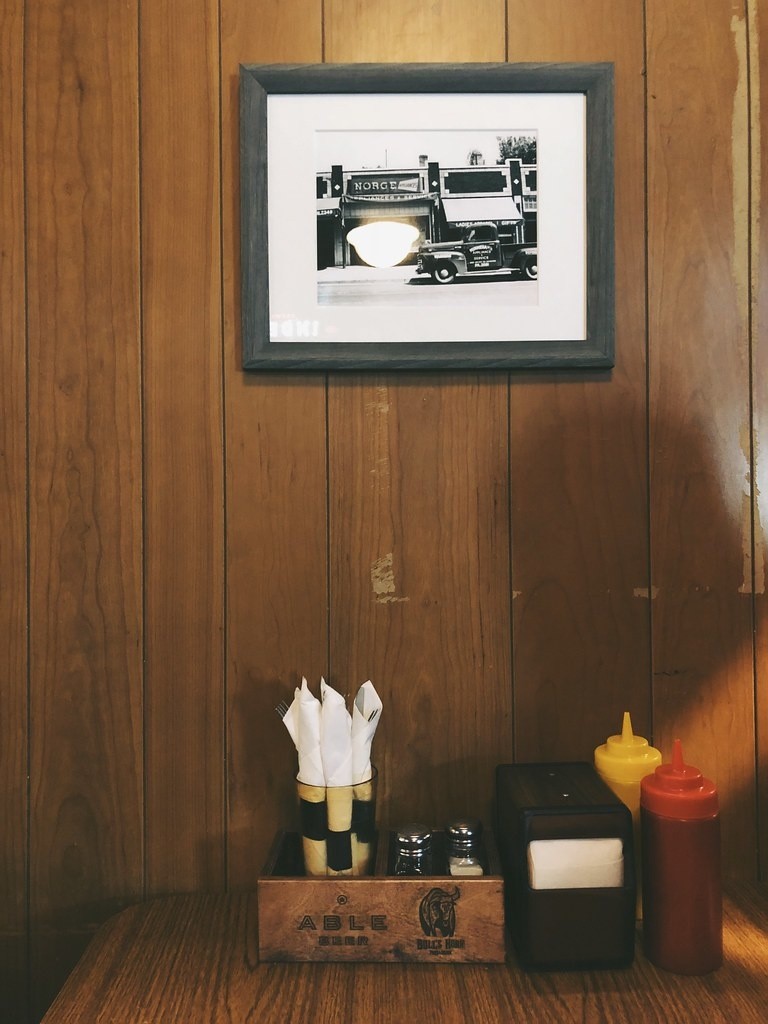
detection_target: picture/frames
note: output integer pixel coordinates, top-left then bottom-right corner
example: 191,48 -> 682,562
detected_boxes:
234,61 -> 618,369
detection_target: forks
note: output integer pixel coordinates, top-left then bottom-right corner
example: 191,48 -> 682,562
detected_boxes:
275,700 -> 289,717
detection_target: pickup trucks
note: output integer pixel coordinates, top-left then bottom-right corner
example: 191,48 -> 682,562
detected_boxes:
413,222 -> 537,281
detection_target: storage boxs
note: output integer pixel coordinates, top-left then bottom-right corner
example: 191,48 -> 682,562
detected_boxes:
256,829 -> 503,965
497,761 -> 634,970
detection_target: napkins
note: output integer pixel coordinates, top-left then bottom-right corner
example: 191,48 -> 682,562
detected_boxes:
282,676 -> 325,803
319,677 -> 354,833
351,680 -> 384,801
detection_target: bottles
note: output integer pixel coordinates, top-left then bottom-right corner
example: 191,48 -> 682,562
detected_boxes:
390,824 -> 433,876
594,712 -> 662,928
641,740 -> 723,974
446,823 -> 481,875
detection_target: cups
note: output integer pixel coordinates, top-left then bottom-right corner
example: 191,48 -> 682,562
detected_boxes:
297,766 -> 377,877
